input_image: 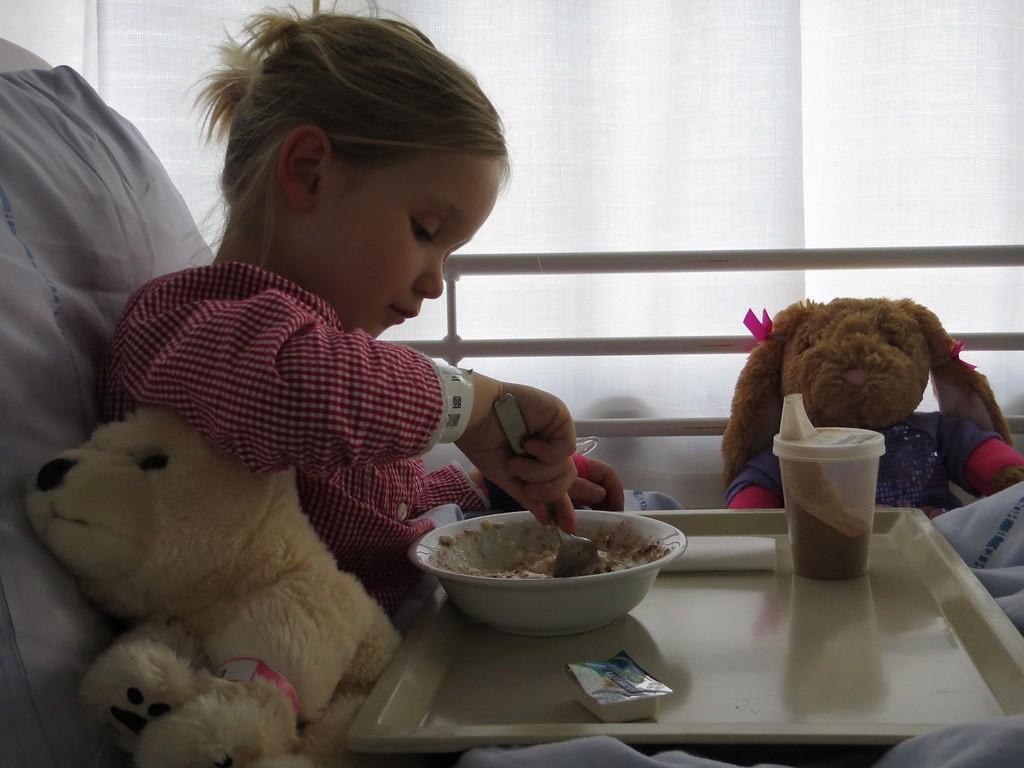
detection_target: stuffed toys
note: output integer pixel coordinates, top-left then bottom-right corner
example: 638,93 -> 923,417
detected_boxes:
722,297 -> 1024,519
24,405 -> 404,768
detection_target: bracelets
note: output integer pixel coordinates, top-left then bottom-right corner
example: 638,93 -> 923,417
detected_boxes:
434,352 -> 475,446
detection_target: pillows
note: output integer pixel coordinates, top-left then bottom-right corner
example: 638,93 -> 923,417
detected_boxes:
0,64 -> 213,768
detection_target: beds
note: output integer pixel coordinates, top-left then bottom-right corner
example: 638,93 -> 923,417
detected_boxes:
0,34 -> 1024,768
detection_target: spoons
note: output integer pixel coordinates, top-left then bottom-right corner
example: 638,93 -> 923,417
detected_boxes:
493,392 -> 598,578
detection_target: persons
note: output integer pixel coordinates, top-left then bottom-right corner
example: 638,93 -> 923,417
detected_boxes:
102,0 -> 625,608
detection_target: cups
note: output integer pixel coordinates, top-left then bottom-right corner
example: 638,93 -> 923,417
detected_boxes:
773,393 -> 886,580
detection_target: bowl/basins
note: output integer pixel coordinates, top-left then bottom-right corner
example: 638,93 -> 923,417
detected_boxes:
409,510 -> 687,636
